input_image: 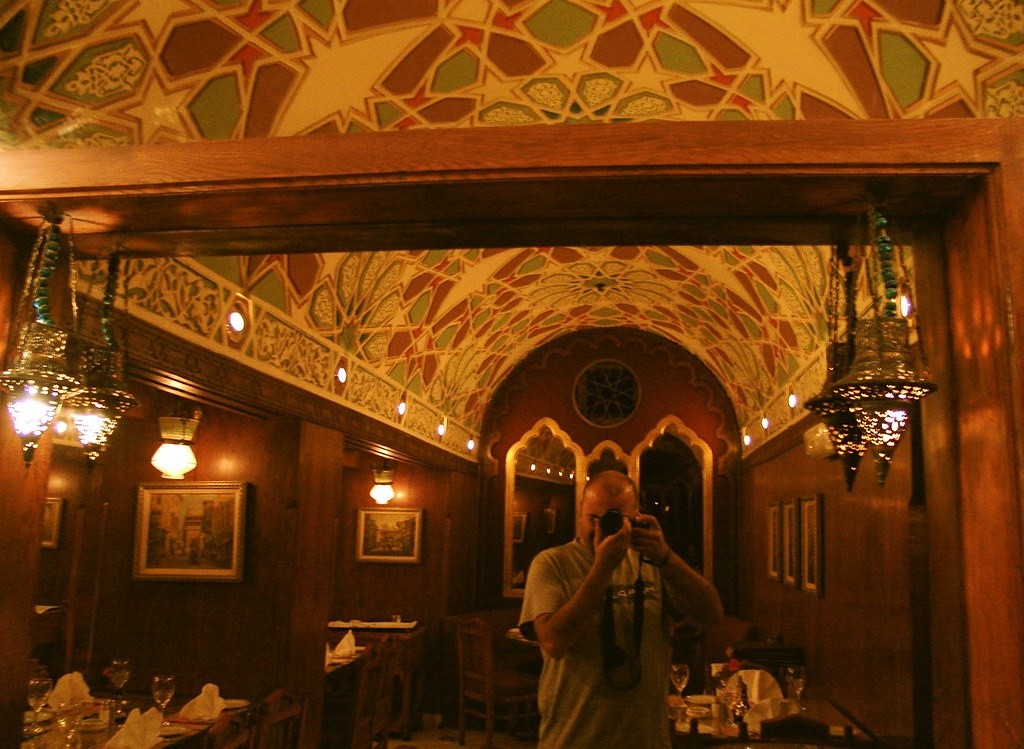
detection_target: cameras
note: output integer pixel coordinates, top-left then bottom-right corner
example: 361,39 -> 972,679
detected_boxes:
599,509 -> 649,545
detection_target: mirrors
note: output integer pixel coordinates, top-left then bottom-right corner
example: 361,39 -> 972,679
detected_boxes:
502,418 -> 715,597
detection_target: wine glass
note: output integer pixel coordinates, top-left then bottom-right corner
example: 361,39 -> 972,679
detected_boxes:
714,677 -> 742,728
786,666 -> 805,702
108,660 -> 130,718
670,663 -> 689,696
27,678 -> 52,734
55,703 -> 80,749
152,675 -> 175,726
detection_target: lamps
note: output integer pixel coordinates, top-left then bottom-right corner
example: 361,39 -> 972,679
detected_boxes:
151,404 -> 200,480
0,209 -> 79,469
60,244 -> 139,474
803,203 -> 869,491
370,465 -> 395,506
822,191 -> 938,487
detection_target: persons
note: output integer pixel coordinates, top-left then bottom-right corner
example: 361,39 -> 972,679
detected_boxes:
518,469 -> 723,749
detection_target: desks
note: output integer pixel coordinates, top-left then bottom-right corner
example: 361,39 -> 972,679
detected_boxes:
667,699 -> 875,749
328,628 -> 429,740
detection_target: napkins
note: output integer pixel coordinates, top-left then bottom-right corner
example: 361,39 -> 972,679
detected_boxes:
179,682 -> 226,721
49,671 -> 94,710
105,706 -> 163,749
334,629 -> 356,658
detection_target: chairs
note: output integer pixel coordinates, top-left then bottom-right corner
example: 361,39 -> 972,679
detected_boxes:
325,635 -> 395,749
760,714 -> 829,745
456,618 -> 539,749
204,689 -> 309,749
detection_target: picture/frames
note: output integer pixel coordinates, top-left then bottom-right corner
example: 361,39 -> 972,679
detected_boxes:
514,511 -> 528,544
355,506 -> 422,563
42,498 -> 63,549
767,493 -> 822,598
132,480 -> 248,583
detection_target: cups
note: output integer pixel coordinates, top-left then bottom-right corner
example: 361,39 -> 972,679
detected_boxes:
78,719 -> 107,749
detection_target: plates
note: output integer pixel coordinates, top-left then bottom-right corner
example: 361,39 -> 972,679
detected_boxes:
159,727 -> 187,736
222,699 -> 251,709
685,694 -> 716,704
